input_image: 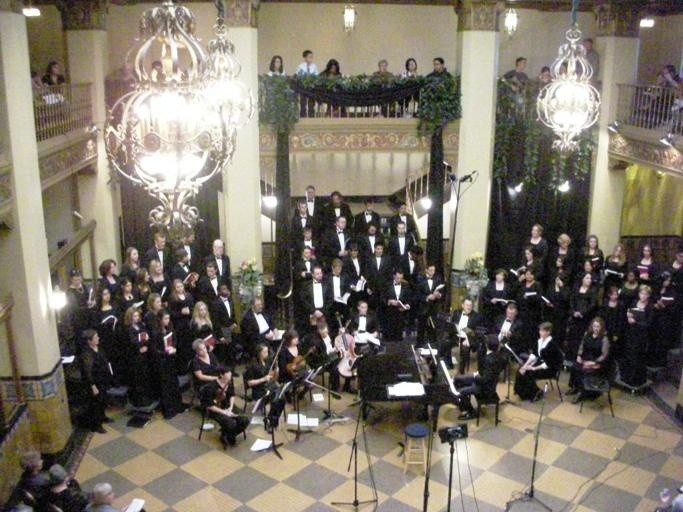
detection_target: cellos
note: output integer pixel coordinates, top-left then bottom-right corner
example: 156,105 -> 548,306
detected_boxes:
334,311 -> 363,378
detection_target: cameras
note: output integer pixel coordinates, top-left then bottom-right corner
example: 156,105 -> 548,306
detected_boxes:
439,422 -> 468,443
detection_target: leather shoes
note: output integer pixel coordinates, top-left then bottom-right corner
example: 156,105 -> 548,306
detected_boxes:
102,417 -> 113,422
531,390 -> 543,400
342,384 -> 358,394
458,411 -> 477,420
571,393 -> 580,404
331,387 -> 341,400
97,425 -> 105,433
565,388 -> 576,395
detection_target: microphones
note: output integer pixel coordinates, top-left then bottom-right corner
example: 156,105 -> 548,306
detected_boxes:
459,170 -> 475,183
544,381 -> 549,392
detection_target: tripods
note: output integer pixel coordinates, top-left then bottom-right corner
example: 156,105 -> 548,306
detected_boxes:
256,376 -> 377,512
505,399 -> 552,512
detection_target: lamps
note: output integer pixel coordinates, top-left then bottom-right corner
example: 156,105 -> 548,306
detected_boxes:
343,4 -> 355,31
659,132 -> 674,146
608,120 -> 621,133
209,0 -> 253,139
22,0 -> 40,18
504,0 -> 519,37
535,0 -> 601,153
103,1 -> 237,247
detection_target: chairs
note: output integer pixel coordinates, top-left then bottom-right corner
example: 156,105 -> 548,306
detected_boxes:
189,319 -> 615,449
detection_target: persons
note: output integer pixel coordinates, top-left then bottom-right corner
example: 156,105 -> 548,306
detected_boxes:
291,186 -> 683,419
65,231 -> 306,454
645,65 -> 683,134
4,451 -> 119,512
31,60 -> 65,98
576,39 -> 599,86
267,49 -> 347,117
501,56 -> 552,119
370,57 -> 452,117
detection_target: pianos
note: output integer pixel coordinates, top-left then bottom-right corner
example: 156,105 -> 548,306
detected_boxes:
357,340 -> 461,433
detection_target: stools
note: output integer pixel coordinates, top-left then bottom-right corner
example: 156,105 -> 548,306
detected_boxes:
400,423 -> 428,476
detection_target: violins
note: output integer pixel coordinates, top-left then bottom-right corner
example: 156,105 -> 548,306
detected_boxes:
286,345 -> 316,376
268,347 -> 280,383
